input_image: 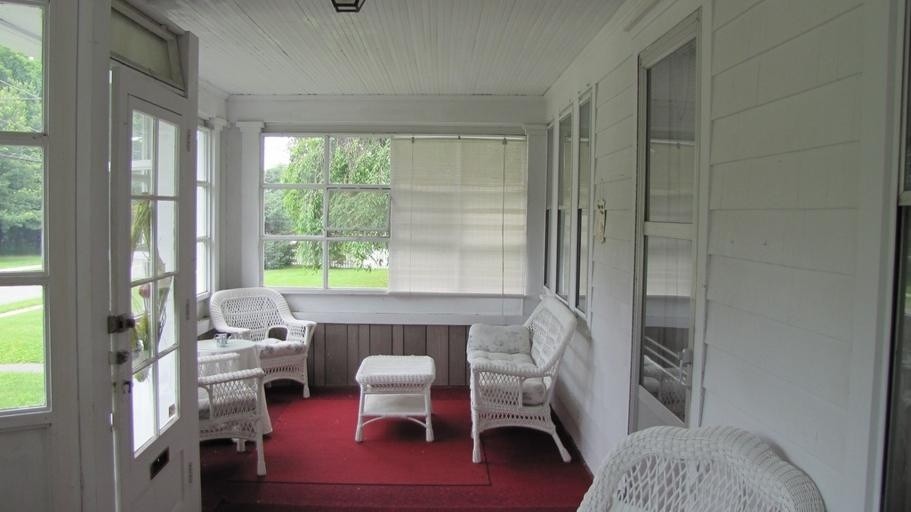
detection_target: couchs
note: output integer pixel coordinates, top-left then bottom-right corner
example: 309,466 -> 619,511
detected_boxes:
462,300 -> 582,466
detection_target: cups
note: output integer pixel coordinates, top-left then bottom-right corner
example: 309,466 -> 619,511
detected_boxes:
215,334 -> 227,347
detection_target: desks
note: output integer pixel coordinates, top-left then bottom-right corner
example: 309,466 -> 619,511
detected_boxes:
191,340 -> 263,443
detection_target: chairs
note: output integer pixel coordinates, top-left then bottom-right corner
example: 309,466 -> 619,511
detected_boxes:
210,287 -> 317,401
189,354 -> 273,486
574,424 -> 827,512
